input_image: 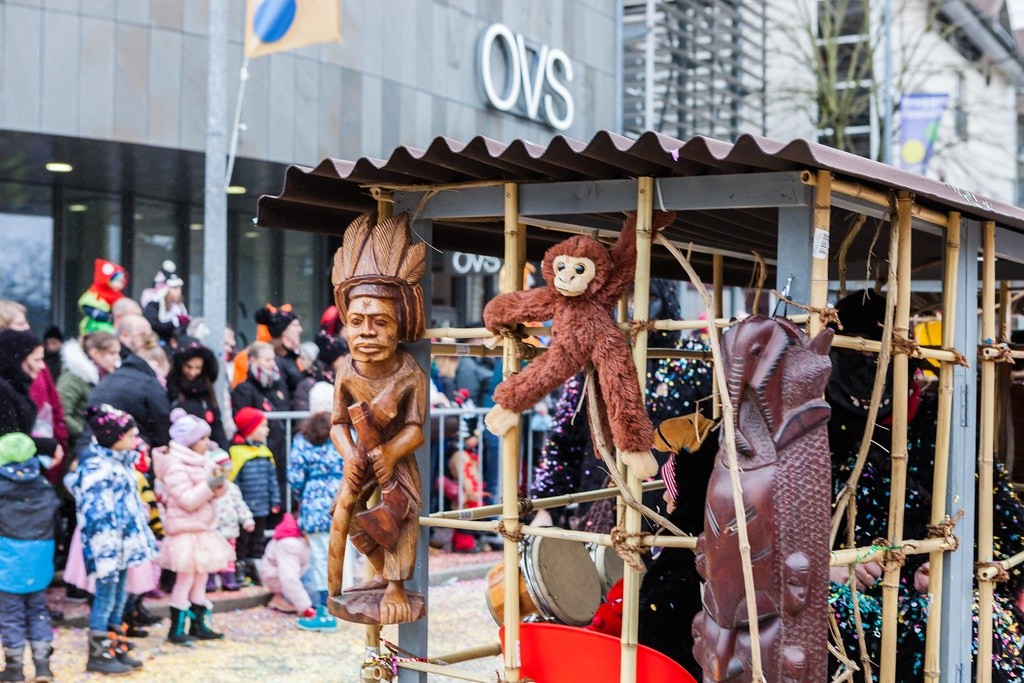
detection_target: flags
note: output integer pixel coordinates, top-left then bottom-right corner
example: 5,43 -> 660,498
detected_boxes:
245,0 -> 343,59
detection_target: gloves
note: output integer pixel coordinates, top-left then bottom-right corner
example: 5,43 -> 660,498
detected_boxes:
172,314 -> 190,328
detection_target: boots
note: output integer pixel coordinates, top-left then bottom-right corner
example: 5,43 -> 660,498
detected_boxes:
189,598 -> 224,639
121,592 -> 163,626
86,630 -> 133,675
296,591 -> 336,633
107,623 -> 143,671
0,647 -> 25,683
160,557 -> 263,592
30,640 -> 53,682
169,606 -> 191,646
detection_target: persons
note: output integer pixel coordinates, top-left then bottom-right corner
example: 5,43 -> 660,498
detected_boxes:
286,409 -> 344,627
638,287 -> 1024,683
0,256 -> 232,603
153,406 -> 225,644
62,401 -> 160,673
326,209 -> 427,628
225,301 -> 350,593
258,499 -> 319,619
0,433 -> 60,683
425,319 -> 565,550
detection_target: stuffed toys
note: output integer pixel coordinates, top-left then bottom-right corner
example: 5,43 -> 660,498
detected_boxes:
481,209 -> 675,480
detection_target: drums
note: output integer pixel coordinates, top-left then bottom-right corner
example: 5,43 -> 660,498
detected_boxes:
483,525 -> 601,628
585,543 -> 644,603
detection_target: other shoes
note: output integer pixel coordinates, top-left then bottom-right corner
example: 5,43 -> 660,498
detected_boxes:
47,606 -> 64,626
268,592 -> 296,613
65,583 -> 94,606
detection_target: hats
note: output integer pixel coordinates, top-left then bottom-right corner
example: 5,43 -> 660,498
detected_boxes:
318,333 -> 349,365
135,436 -> 150,453
268,309 -> 300,338
168,407 -> 211,447
154,260 -> 184,287
234,407 -> 264,438
0,432 -> 37,467
111,271 -> 123,281
308,381 -> 335,415
85,404 -> 136,447
45,327 -> 63,342
0,329 -> 41,367
